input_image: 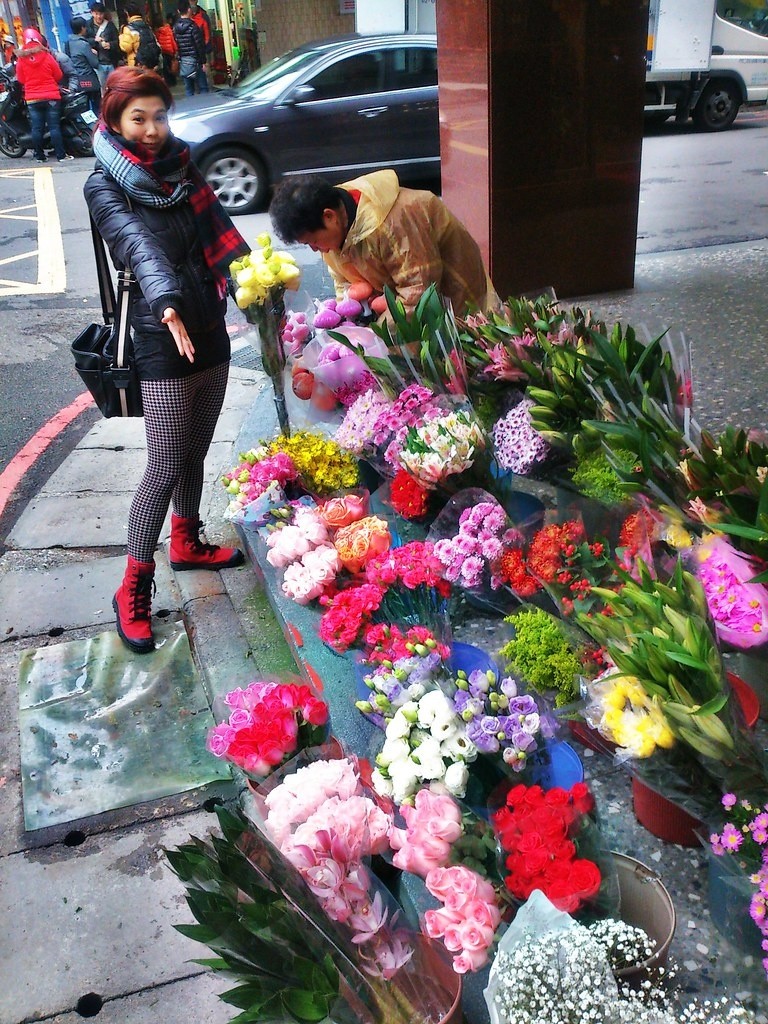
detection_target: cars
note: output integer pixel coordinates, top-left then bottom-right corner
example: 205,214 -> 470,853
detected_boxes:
165,32 -> 441,216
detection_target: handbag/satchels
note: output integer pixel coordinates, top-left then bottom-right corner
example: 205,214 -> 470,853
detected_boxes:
179,57 -> 199,80
69,318 -> 141,416
302,326 -> 389,411
76,74 -> 100,92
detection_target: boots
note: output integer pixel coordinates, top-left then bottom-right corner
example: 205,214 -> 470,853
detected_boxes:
169,512 -> 245,571
112,554 -> 156,653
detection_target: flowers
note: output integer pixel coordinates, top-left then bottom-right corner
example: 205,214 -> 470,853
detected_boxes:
162,233 -> 767,1024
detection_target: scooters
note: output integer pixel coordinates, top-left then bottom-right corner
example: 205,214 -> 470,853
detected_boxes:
0,57 -> 98,158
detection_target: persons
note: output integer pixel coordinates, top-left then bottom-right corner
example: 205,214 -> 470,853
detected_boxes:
16,29 -> 74,163
64,17 -> 101,119
172,0 -> 209,96
40,35 -> 78,96
189,0 -> 213,94
84,2 -> 119,99
152,13 -> 177,87
119,3 -> 161,71
271,168 -> 499,320
84,66 -> 259,654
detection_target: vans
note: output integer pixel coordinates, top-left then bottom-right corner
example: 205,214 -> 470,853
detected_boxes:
355,0 -> 768,133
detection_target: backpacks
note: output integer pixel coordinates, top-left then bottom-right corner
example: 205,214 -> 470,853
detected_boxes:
129,21 -> 160,68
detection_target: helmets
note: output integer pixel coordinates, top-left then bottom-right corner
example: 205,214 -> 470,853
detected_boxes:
22,29 -> 42,44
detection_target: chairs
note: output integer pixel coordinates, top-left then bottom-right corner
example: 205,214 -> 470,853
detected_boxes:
343,54 -> 377,95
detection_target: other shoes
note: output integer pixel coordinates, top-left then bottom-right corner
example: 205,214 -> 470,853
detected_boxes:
59,154 -> 74,162
37,156 -> 49,162
